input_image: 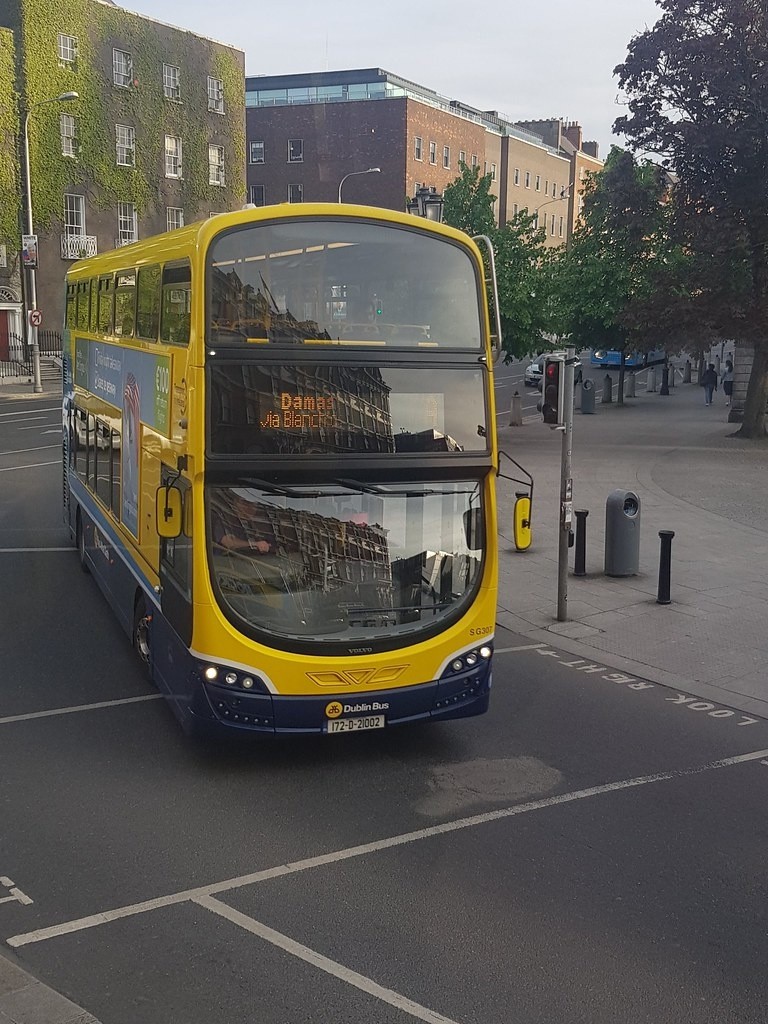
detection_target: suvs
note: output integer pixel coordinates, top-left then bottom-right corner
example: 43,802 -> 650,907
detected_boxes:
525,353 -> 584,389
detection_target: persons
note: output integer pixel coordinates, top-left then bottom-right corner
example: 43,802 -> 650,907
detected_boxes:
213,493 -> 277,555
355,301 -> 375,323
700,364 -> 717,406
720,360 -> 733,406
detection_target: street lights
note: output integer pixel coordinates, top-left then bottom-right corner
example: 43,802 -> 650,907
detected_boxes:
22,92 -> 83,391
338,167 -> 381,205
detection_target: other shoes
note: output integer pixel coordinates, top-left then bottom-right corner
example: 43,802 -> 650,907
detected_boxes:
710,400 -> 714,403
705,403 -> 709,406
725,402 -> 729,406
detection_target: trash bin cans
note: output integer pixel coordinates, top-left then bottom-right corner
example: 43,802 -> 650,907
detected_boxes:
581,378 -> 595,414
604,489 -> 640,576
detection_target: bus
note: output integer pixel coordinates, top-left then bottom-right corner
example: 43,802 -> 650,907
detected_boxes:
60,202 -> 534,732
589,340 -> 670,369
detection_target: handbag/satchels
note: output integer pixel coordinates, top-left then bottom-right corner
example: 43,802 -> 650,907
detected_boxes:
699,370 -> 709,386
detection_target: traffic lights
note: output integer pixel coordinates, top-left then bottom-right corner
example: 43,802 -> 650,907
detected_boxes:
537,355 -> 564,425
